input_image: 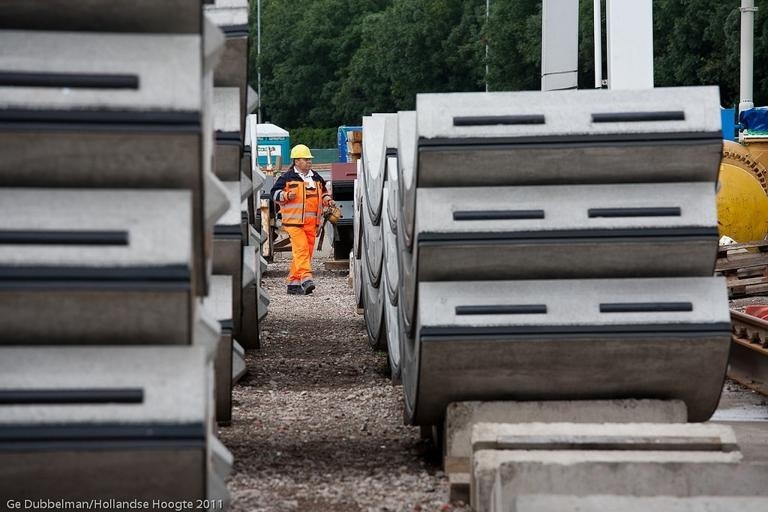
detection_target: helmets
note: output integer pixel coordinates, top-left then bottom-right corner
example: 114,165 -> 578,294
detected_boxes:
289,144 -> 313,159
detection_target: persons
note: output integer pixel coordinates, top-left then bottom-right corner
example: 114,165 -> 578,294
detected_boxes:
268,143 -> 338,296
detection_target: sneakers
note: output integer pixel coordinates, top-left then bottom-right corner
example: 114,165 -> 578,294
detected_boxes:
287,284 -> 305,295
303,280 -> 315,294
